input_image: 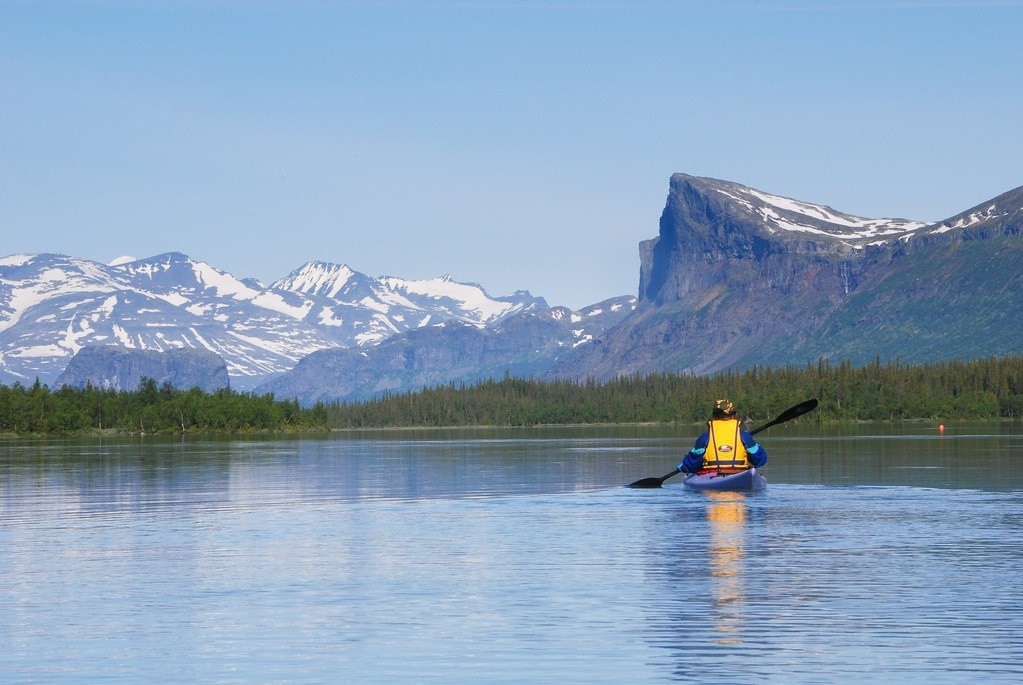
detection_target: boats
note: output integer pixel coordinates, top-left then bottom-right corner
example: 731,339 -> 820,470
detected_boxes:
683,467 -> 767,492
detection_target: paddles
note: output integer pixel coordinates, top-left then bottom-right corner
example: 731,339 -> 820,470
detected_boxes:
627,397 -> 820,488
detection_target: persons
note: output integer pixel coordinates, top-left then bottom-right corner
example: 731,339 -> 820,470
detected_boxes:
680,399 -> 768,476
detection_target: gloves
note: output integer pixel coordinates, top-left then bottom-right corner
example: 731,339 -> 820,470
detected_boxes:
681,466 -> 686,473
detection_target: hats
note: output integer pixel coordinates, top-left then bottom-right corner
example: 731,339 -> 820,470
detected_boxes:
713,398 -> 735,414
